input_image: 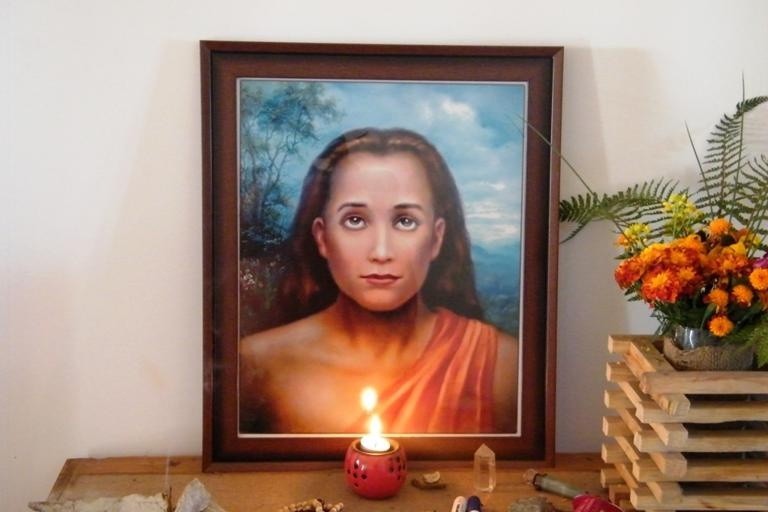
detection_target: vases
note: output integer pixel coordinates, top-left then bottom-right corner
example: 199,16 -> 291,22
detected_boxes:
659,318 -> 755,373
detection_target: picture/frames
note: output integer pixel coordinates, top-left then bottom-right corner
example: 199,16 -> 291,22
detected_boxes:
195,40 -> 570,474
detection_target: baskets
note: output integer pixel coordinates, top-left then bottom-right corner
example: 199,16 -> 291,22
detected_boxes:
663,336 -> 753,370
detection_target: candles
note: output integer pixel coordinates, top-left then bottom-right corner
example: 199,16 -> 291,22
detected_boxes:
354,430 -> 394,459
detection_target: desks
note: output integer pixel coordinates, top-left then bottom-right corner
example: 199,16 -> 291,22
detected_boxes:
37,456 -> 636,512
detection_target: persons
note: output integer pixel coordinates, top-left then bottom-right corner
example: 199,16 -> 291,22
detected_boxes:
239,129 -> 518,435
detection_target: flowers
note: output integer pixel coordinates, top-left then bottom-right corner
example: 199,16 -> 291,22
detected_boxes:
513,73 -> 768,368
